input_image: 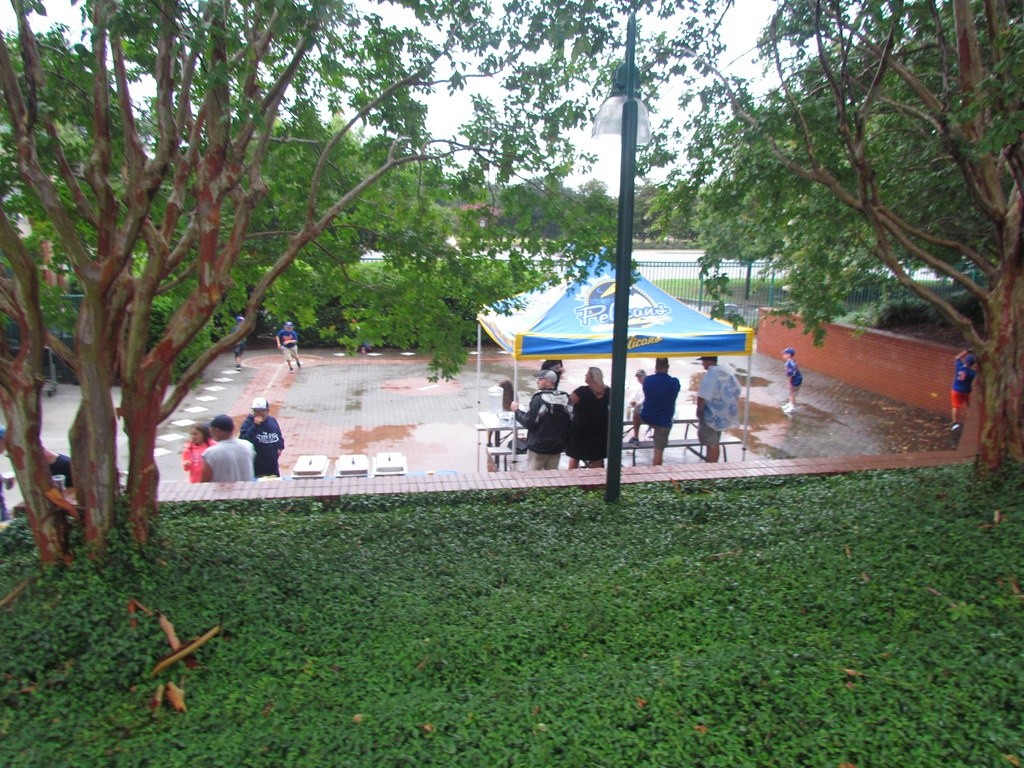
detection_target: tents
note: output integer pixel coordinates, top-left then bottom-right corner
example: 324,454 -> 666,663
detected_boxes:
474,236 -> 755,472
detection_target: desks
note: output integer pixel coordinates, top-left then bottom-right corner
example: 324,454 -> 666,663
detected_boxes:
476,401 -> 705,469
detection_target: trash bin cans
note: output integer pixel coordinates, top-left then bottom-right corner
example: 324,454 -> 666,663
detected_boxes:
290,452 -> 408,478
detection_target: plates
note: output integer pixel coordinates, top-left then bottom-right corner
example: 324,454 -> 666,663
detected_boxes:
498,412 -> 514,419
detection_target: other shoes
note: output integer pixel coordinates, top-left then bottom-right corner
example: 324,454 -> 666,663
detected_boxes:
297,362 -> 300,367
785,407 -> 797,413
781,404 -> 790,409
629,438 -> 639,447
236,365 -> 241,370
290,367 -> 293,372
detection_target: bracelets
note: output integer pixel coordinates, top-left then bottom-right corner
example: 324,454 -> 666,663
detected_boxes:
966,349 -> 971,352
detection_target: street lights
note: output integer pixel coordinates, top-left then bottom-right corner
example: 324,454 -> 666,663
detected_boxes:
591,13 -> 649,502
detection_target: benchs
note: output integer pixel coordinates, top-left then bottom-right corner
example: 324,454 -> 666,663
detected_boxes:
487,435 -> 742,471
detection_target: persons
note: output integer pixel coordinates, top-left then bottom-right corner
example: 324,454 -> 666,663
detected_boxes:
511,369 -> 574,470
181,423 -> 218,483
238,397 -> 284,477
275,321 -> 301,372
630,354 -> 681,466
0,426 -> 74,522
780,347 -> 803,413
950,349 -> 978,431
231,316 -> 246,371
631,368 -> 646,411
199,414 -> 257,482
566,367 -> 611,469
542,360 -> 564,389
351,319 -> 368,354
695,357 -> 743,462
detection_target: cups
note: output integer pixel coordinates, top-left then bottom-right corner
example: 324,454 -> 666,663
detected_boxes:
3,472 -> 15,488
52,474 -> 66,492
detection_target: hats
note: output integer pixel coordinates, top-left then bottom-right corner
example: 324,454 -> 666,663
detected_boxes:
635,370 -> 645,376
781,349 -> 794,354
697,357 -> 717,361
250,398 -> 269,409
285,322 -> 293,327
204,414 -> 234,430
534,369 -> 557,382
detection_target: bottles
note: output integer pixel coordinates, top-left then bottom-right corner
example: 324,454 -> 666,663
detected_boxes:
626,406 -> 631,419
